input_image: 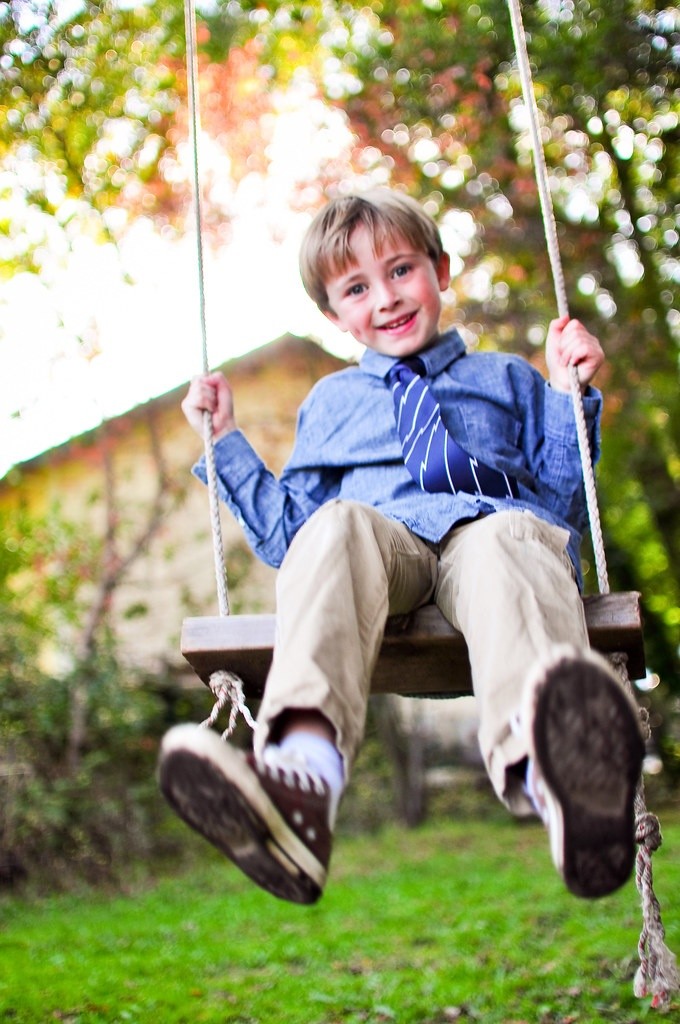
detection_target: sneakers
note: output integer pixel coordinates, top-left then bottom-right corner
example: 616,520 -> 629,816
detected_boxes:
524,645 -> 643,900
155,724 -> 339,905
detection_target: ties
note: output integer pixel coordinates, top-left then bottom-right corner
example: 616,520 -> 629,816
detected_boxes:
384,361 -> 519,501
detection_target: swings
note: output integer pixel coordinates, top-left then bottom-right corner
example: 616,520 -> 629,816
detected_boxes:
177,0 -> 648,698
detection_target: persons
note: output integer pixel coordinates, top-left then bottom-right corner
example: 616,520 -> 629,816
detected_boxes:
158,193 -> 648,905
